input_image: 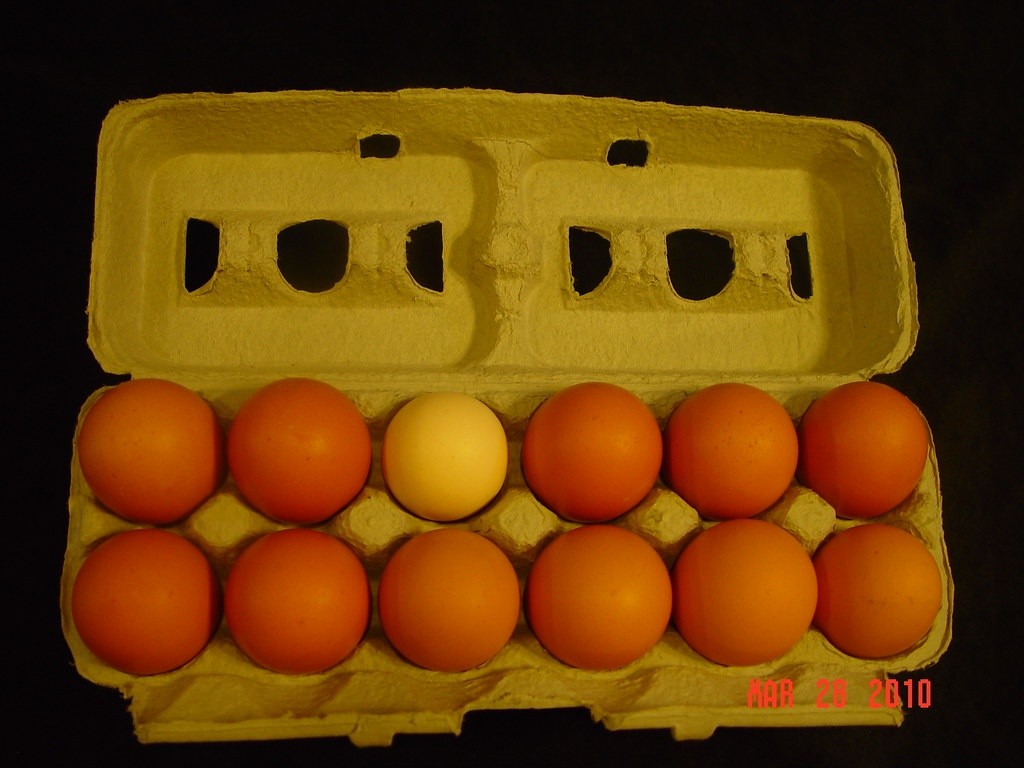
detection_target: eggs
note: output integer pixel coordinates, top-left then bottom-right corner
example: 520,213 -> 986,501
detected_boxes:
73,379 -> 942,676
